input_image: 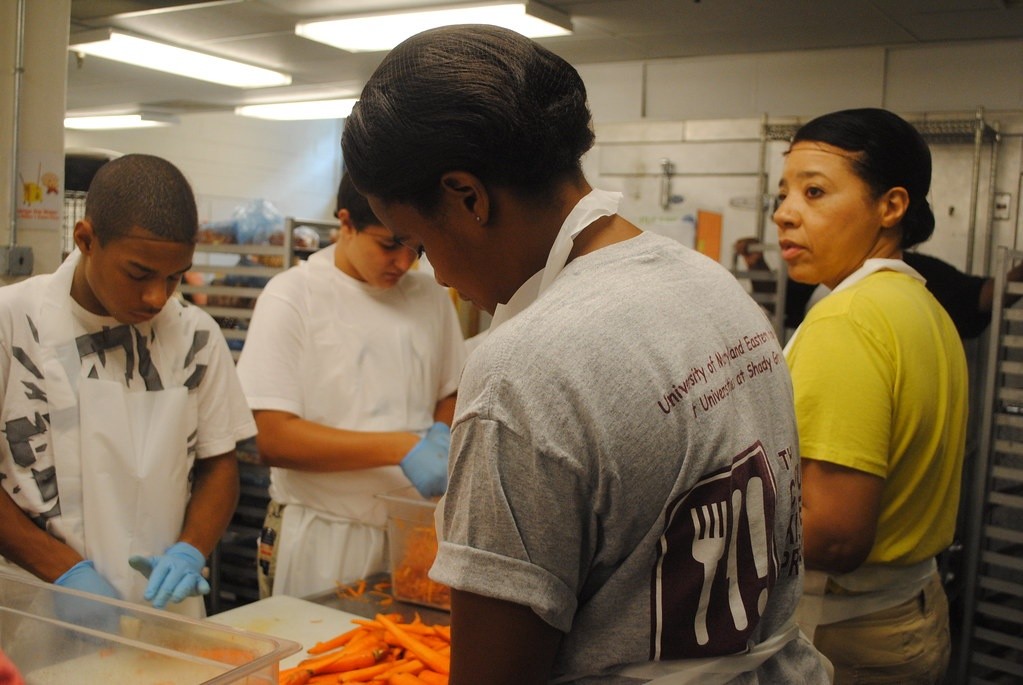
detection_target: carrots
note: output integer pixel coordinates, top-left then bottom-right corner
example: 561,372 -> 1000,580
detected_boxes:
200,579 -> 450,685
392,523 -> 450,609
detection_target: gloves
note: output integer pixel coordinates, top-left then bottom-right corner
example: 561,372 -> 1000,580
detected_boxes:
52,560 -> 141,645
400,421 -> 452,500
129,542 -> 210,609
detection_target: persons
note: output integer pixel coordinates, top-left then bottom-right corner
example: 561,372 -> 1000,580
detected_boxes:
338,24 -> 799,685
733,106 -> 1023,685
0,155 -> 259,647
234,175 -> 465,600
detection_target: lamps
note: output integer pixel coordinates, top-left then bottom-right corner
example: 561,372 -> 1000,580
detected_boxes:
67,26 -> 294,92
295,0 -> 573,56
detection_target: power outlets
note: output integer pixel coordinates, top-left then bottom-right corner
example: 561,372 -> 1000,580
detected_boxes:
993,192 -> 1011,219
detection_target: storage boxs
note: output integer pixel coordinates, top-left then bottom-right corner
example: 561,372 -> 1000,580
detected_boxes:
0,574 -> 303,685
372,483 -> 451,610
